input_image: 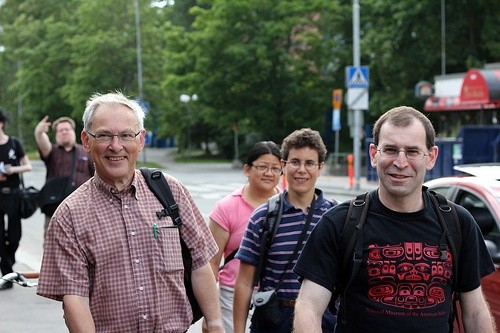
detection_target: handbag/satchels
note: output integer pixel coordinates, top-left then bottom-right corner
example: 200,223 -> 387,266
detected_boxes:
16,187 -> 39,219
140,167 -> 204,324
250,289 -> 282,327
38,176 -> 75,217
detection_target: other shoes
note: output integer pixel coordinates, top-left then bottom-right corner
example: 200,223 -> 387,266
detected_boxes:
0,277 -> 13,289
13,272 -> 28,287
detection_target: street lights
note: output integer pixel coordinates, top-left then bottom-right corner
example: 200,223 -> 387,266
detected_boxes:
180,93 -> 198,160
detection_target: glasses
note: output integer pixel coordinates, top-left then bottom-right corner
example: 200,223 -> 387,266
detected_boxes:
282,159 -> 321,170
252,164 -> 282,176
86,130 -> 141,144
375,145 -> 430,160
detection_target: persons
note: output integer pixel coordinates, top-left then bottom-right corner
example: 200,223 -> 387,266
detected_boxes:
293,106 -> 497,333
203,141 -> 284,333
0,107 -> 32,288
34,115 -> 95,250
36,88 -> 226,333
233,128 -> 341,333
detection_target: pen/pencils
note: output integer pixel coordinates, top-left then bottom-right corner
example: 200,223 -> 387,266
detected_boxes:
153,223 -> 158,239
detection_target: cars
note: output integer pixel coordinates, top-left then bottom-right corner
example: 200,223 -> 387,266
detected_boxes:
420,162 -> 500,333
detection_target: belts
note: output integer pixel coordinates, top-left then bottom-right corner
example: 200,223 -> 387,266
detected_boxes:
276,299 -> 295,307
